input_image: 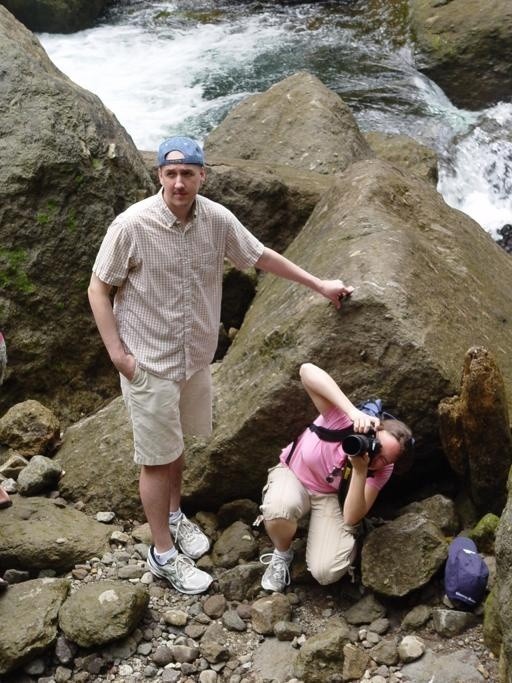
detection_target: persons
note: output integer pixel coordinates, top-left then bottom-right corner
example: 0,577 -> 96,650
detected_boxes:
253,361 -> 417,593
85,134 -> 355,595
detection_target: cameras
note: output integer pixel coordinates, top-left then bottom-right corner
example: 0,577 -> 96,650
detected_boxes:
342,427 -> 381,459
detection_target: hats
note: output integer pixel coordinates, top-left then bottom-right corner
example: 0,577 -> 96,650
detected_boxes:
445,535 -> 489,611
155,137 -> 206,168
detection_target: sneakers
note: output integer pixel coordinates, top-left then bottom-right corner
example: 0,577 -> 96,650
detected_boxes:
261,548 -> 294,594
146,543 -> 214,596
168,511 -> 211,560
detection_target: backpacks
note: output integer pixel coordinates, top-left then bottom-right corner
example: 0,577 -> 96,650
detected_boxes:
307,399 -> 396,443
